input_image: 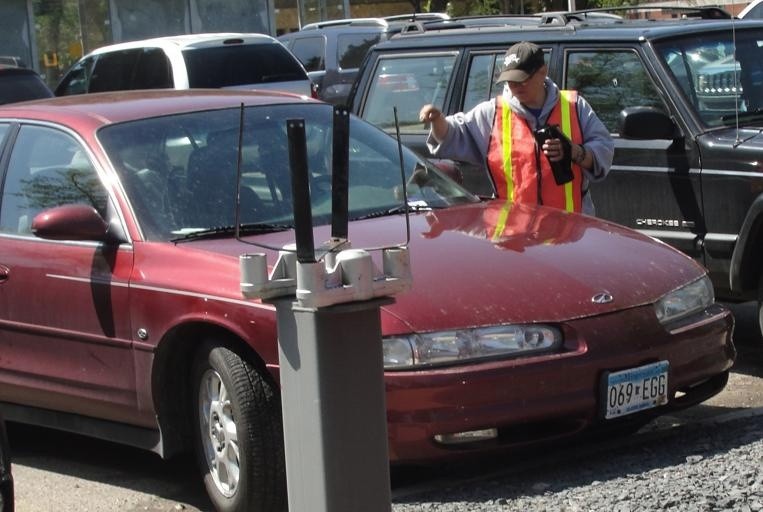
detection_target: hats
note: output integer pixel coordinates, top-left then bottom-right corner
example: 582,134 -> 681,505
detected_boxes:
495,41 -> 544,85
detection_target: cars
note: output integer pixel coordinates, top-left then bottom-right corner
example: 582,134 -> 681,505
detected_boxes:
0,88 -> 738,512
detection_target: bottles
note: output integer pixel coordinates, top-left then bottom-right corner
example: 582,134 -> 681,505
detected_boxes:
532,123 -> 574,187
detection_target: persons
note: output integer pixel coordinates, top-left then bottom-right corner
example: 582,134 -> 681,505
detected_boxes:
391,166 -> 590,252
419,39 -> 615,218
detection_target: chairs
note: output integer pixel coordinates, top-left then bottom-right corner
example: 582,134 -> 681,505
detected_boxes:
15,139 -> 270,240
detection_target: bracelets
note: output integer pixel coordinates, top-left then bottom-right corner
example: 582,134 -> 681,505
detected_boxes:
579,142 -> 586,164
